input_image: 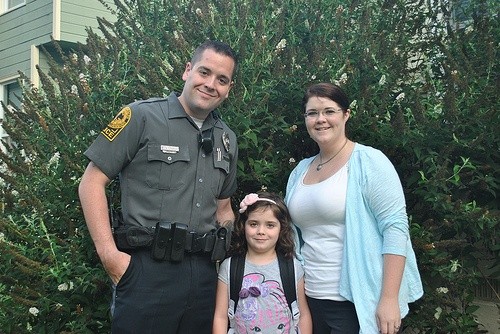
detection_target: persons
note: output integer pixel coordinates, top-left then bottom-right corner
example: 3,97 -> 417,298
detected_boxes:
284,82 -> 424,334
212,192 -> 313,334
78,40 -> 239,334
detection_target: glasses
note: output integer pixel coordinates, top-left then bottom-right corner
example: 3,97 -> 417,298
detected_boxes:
303,108 -> 344,118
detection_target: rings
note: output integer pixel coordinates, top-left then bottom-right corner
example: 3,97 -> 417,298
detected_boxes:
394,325 -> 401,329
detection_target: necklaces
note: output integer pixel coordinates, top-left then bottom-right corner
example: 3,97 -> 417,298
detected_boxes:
316,136 -> 348,171
